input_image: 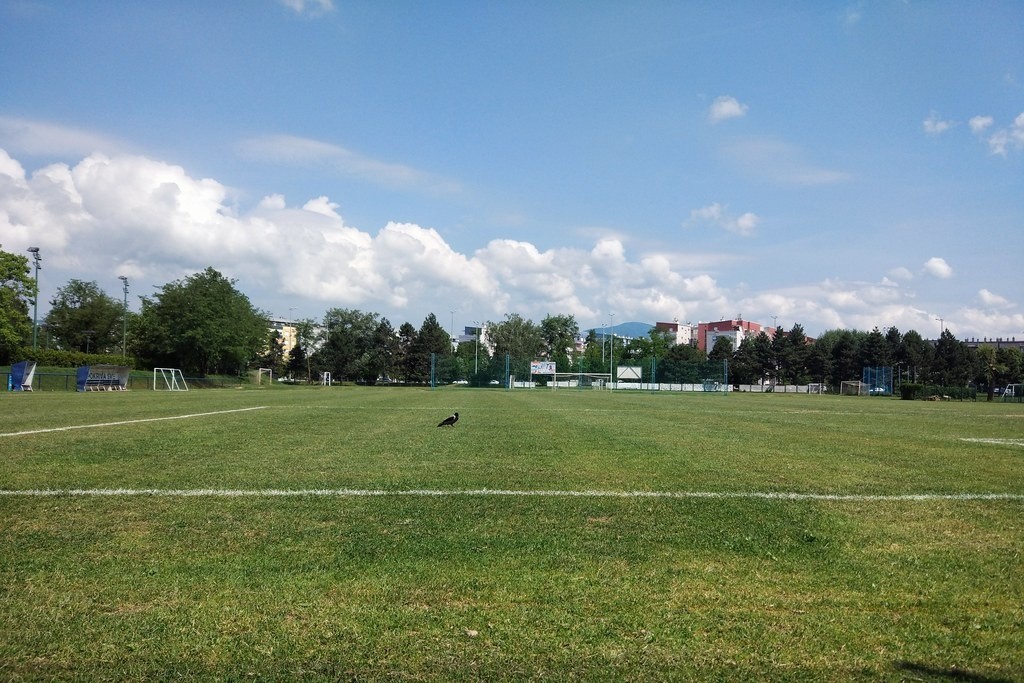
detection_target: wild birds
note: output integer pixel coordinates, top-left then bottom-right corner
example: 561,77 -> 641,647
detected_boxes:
437,412 -> 460,428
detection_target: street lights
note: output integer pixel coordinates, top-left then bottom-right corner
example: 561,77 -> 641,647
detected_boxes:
118,276 -> 129,366
936,317 -> 944,337
449,309 -> 457,355
26,246 -> 42,356
602,323 -> 608,366
608,313 -> 615,394
473,319 -> 480,374
289,306 -> 297,350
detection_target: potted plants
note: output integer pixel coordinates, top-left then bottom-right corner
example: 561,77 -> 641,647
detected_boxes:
900,383 -> 922,400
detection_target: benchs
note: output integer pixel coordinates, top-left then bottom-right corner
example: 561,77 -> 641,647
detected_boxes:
22,384 -> 33,392
86,379 -> 122,392
